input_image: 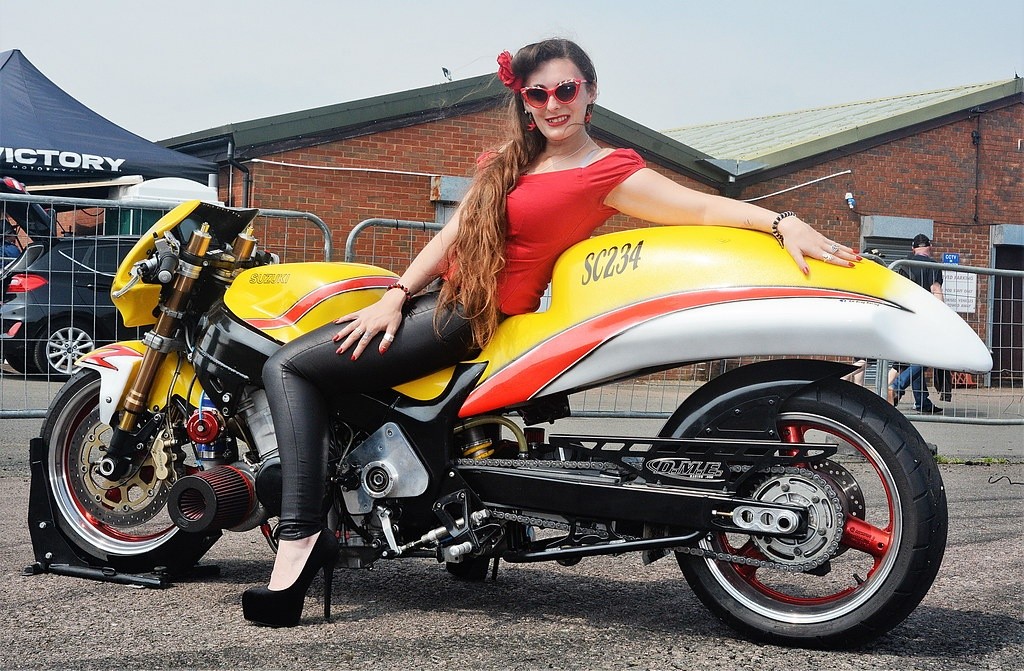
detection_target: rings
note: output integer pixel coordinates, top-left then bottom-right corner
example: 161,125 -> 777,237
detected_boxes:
355,328 -> 363,335
383,333 -> 394,342
831,244 -> 839,255
823,253 -> 833,263
363,332 -> 373,338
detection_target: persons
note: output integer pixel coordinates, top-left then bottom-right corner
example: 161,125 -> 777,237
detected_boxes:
888,233 -> 944,413
242,38 -> 863,630
841,247 -> 883,385
0,230 -> 22,270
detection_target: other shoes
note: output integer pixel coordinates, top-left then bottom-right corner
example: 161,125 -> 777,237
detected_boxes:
917,403 -> 943,414
888,385 -> 901,407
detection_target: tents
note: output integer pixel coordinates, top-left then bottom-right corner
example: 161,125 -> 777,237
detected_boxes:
0,49 -> 218,213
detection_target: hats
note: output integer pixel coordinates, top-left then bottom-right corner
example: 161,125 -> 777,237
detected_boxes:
911,234 -> 931,248
863,247 -> 886,258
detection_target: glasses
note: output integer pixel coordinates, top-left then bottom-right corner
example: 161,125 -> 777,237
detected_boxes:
520,78 -> 587,109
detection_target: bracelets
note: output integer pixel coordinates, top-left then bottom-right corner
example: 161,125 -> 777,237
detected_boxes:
772,211 -> 796,241
386,283 -> 411,304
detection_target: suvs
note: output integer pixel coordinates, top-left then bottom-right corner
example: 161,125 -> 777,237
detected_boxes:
0,234 -> 173,382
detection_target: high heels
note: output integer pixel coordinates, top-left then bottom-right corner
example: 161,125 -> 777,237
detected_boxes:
242,527 -> 339,627
446,555 -> 500,582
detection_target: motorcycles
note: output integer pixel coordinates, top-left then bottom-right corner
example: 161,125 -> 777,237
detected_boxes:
20,199 -> 994,651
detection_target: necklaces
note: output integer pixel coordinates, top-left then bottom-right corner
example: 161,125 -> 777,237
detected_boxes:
526,135 -> 590,174
